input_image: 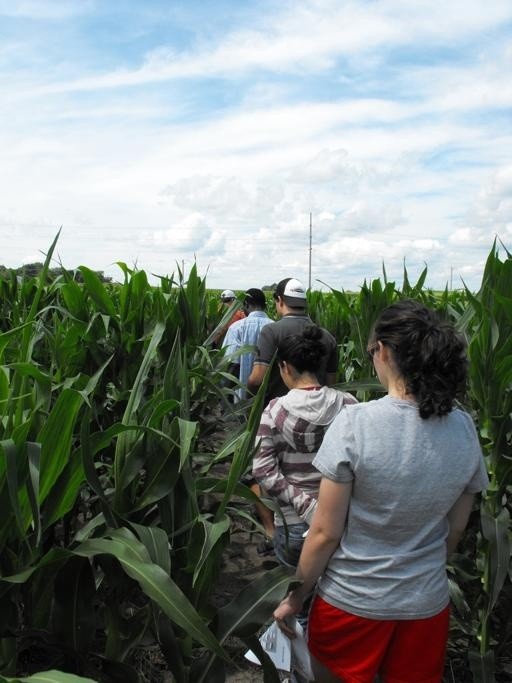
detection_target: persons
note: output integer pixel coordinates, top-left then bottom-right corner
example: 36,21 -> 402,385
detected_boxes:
220,288 -> 275,425
273,299 -> 491,683
212,289 -> 246,349
251,325 -> 359,683
246,278 -> 339,558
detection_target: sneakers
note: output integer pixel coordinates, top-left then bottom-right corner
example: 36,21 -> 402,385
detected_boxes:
256,535 -> 276,558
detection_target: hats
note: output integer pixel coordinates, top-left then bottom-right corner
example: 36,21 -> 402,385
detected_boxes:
221,289 -> 239,299
276,277 -> 309,310
